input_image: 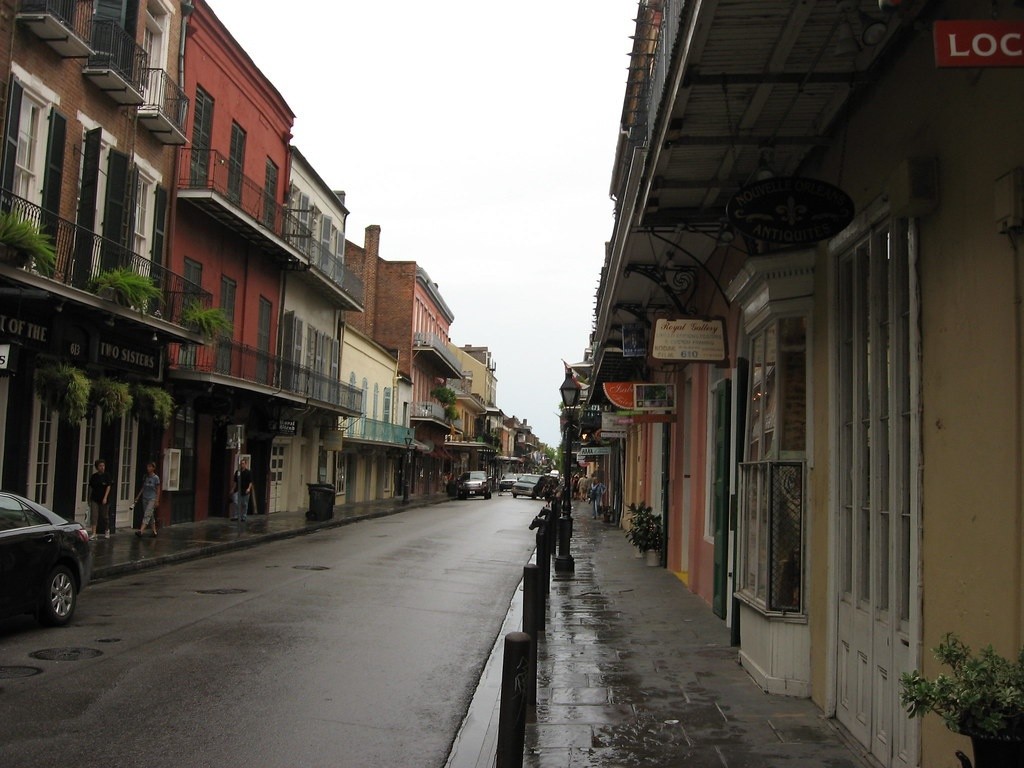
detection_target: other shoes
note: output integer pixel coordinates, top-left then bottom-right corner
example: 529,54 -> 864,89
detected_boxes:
149,532 -> 159,538
241,519 -> 245,522
592,516 -> 596,519
230,518 -> 238,521
135,531 -> 144,537
597,516 -> 600,519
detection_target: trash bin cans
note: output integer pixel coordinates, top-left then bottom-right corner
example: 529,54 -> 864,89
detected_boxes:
447,480 -> 456,497
305,483 -> 335,522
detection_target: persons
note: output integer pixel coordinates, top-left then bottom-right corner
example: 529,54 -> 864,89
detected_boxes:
134,462 -> 161,538
88,459 -> 112,541
570,475 -> 607,519
229,459 -> 253,521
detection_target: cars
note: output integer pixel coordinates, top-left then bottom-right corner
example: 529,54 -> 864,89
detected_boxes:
1,491 -> 90,627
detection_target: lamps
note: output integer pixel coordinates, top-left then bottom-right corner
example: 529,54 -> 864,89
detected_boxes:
856,7 -> 888,47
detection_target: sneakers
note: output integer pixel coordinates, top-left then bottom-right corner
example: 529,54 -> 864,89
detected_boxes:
89,534 -> 98,541
104,529 -> 110,539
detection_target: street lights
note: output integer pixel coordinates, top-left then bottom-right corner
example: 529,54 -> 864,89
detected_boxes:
553,368 -> 582,581
401,429 -> 413,505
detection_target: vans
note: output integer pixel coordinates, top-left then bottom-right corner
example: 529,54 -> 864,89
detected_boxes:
511,474 -> 557,500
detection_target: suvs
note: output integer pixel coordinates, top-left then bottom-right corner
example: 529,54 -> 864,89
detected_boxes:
499,473 -> 518,491
457,470 -> 492,500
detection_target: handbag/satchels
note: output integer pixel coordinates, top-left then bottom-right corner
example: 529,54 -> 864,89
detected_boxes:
85,506 -> 92,528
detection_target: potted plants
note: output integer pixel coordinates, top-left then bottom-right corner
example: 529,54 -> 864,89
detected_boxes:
622,500 -> 643,558
632,500 -> 664,567
0,207 -> 241,431
896,632 -> 1024,768
